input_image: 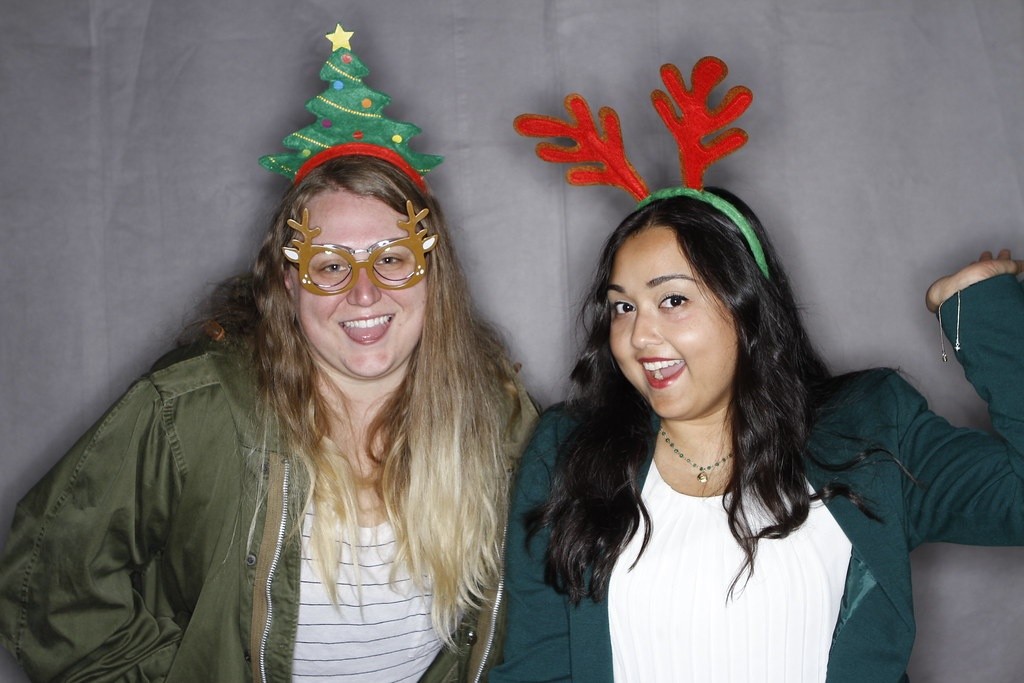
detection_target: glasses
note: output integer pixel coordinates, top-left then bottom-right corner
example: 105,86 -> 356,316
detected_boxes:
281,200 -> 439,296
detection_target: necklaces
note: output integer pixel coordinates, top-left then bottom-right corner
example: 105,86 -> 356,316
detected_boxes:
660,424 -> 733,482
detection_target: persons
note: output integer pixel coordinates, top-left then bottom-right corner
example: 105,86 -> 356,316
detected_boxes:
487,56 -> 1023,683
0,22 -> 537,683
